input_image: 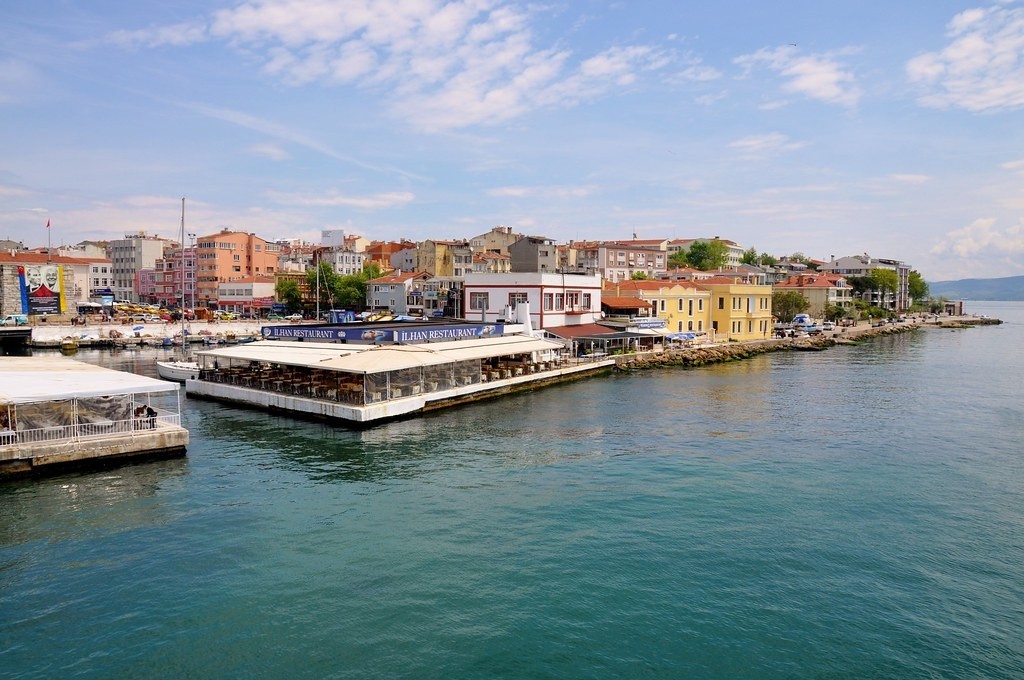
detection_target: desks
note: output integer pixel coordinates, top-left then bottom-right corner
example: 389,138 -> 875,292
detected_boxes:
0,407 -> 157,442
198,353 -> 561,405
561,336 -> 703,367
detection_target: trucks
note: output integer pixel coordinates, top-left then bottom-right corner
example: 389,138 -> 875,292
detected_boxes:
1,314 -> 29,326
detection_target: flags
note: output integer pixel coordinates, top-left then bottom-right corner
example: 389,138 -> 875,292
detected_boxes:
46,220 -> 50,228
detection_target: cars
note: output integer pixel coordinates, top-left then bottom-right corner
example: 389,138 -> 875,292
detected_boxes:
117,299 -> 304,323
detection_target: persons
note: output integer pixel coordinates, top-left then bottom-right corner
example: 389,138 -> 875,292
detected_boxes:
45,267 -> 59,292
71,307 -> 118,326
146,407 -> 157,428
577,343 -> 586,357
26,268 -> 42,293
134,404 -> 147,427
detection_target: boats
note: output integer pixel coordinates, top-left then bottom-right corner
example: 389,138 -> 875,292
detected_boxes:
153,359 -> 214,382
61,338 -> 79,351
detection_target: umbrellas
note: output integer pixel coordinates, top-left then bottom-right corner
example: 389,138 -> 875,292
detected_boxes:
666,332 -> 696,345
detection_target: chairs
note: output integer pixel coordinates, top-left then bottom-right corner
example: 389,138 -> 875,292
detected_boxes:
117,400 -> 158,426
198,358 -> 562,403
